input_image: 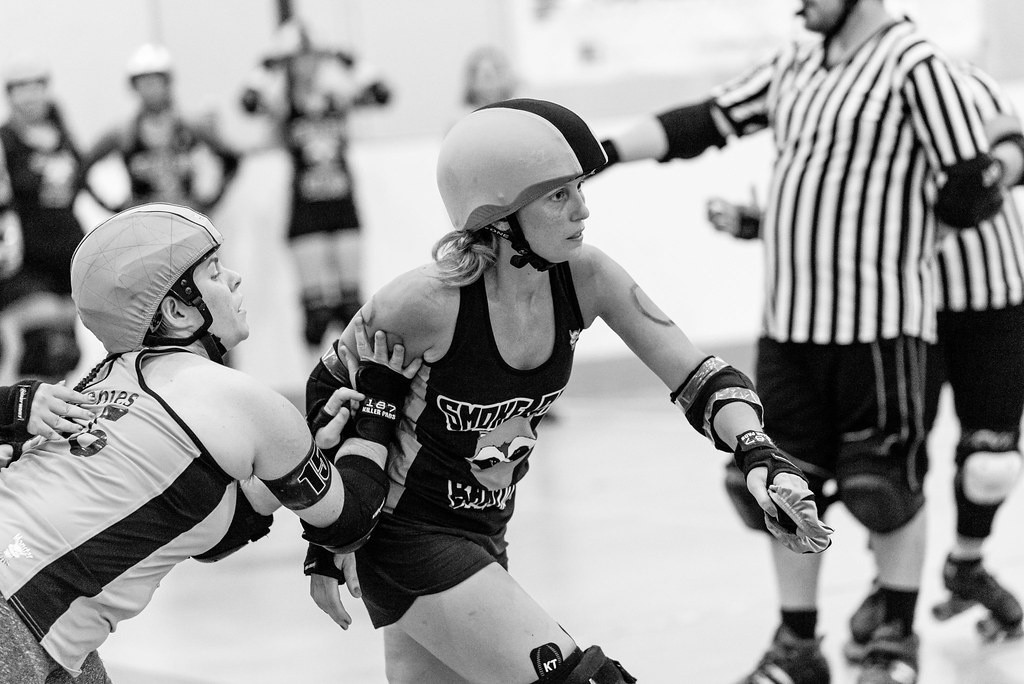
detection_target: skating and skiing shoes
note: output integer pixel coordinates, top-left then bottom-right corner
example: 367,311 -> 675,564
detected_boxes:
933,553 -> 1023,643
843,580 -> 879,662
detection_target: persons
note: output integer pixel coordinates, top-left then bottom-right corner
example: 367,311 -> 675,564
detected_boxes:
597,1 -> 1023,684
304,99 -> 836,684
0,14 -> 423,684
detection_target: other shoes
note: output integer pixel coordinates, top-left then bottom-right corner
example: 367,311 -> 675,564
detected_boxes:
859,628 -> 922,682
740,625 -> 832,684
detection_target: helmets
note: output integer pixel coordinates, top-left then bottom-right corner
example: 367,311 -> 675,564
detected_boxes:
70,202 -> 222,352
127,43 -> 172,83
5,60 -> 49,89
439,98 -> 609,233
